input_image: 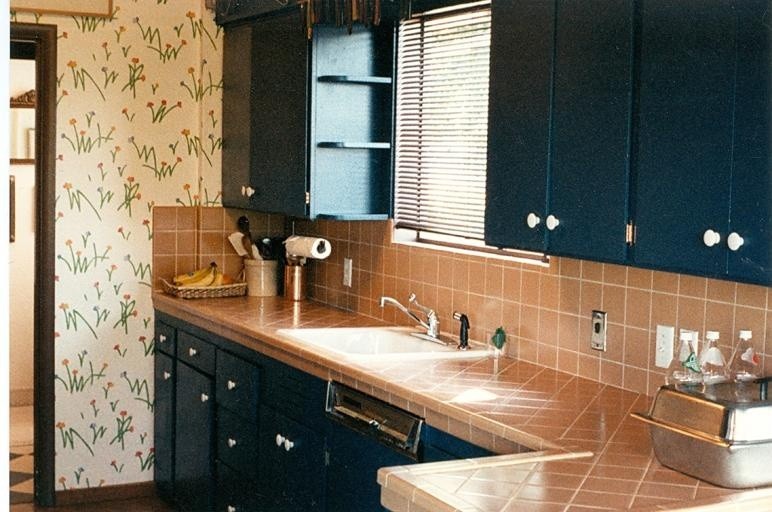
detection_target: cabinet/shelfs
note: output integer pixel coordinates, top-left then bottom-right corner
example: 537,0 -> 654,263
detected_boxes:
309,20 -> 396,221
633,0 -> 772,287
153,308 -> 498,512
218,0 -> 309,217
484,0 -> 637,266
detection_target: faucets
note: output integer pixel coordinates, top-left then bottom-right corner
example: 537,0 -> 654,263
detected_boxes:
379,293 -> 439,337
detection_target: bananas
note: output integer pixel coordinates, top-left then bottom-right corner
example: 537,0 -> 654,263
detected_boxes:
173,262 -> 223,287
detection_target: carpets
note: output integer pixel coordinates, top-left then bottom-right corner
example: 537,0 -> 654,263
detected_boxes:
9,445 -> 35,505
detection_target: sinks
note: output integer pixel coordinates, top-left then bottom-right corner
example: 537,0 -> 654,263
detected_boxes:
276,325 -> 493,361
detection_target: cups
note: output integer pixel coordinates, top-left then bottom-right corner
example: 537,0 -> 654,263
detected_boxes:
283,265 -> 307,303
245,259 -> 279,297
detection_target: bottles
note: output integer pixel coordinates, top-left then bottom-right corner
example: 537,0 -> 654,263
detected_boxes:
663,327 -> 763,392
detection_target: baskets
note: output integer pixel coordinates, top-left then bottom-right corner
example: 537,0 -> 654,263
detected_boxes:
159,270 -> 247,299
